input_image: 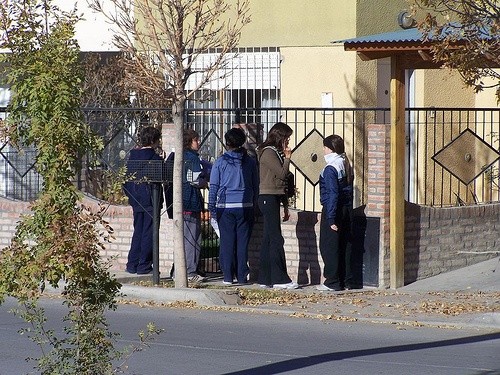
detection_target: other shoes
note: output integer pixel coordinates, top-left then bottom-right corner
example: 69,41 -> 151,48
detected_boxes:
239,280 -> 252,285
258,283 -> 272,288
148,270 -> 160,274
273,282 -> 299,289
316,284 -> 335,291
222,280 -> 232,284
187,274 -> 209,283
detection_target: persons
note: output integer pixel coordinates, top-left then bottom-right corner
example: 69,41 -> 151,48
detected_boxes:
255,122 -> 299,290
124,126 -> 165,275
164,129 -> 214,282
317,135 -> 358,292
209,127 -> 258,286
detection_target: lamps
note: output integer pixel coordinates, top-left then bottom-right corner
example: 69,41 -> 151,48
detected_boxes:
399,10 -> 417,29
